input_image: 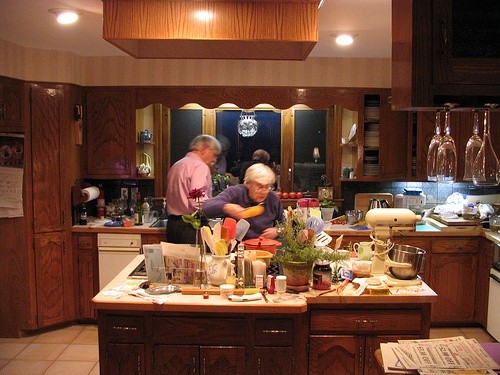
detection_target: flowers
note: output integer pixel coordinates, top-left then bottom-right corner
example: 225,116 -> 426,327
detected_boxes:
182,186 -> 205,230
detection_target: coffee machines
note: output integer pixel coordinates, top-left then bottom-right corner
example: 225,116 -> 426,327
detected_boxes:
394,188 -> 427,225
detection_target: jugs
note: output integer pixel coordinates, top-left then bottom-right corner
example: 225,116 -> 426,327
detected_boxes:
353,242 -> 373,261
411,208 -> 423,216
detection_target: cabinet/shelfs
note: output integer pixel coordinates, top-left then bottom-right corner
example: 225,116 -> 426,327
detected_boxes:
307,305 -> 432,375
0,75 -> 73,338
74,233 -> 98,320
329,235 -> 494,330
98,309 -> 308,375
358,88 -> 500,183
339,143 -> 357,182
136,141 -> 155,180
84,89 -> 132,177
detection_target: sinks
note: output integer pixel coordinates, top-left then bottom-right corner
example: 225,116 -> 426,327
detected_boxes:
149,219 -> 168,228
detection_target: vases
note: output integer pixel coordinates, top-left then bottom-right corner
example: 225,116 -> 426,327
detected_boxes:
193,227 -> 209,290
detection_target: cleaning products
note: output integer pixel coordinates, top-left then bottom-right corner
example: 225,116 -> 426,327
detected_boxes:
134,202 -> 145,226
160,197 -> 169,219
143,198 -> 149,212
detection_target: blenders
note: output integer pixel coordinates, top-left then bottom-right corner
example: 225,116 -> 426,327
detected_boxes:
364,208 -> 423,286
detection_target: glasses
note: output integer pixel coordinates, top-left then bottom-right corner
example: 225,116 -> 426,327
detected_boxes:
250,181 -> 275,192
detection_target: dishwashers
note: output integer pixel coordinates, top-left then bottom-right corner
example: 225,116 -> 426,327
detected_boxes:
97,232 -> 141,291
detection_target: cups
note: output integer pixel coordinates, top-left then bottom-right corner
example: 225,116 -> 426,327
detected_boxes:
208,255 -> 232,285
345,210 -> 364,224
489,214 -> 500,232
461,207 -> 477,219
220,284 -> 234,299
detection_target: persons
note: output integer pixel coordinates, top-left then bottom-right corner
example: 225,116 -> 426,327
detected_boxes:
201,162 -> 287,257
166,135 -> 221,252
215,134 -> 278,191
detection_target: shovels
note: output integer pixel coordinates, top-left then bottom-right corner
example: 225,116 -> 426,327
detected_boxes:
200,217 -> 250,255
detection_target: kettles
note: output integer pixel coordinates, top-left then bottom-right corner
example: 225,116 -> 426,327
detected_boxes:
368,198 -> 391,211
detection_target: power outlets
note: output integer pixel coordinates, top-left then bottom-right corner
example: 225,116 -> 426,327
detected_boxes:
121,188 -> 128,201
130,188 -> 138,200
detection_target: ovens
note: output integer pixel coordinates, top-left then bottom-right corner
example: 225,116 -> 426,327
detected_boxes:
487,243 -> 500,344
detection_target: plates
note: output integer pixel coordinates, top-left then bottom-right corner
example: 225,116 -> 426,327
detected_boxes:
104,222 -> 122,227
146,284 -> 179,294
364,99 -> 417,176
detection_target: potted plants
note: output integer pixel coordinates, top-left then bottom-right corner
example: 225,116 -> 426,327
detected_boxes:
269,207 -> 349,286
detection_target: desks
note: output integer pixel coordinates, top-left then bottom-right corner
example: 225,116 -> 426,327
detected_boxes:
374,343 -> 500,375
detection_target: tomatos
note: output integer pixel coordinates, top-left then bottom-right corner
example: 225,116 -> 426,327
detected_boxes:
275,192 -> 304,199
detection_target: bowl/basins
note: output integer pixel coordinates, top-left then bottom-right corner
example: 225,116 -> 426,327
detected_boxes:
240,237 -> 282,270
389,244 -> 427,280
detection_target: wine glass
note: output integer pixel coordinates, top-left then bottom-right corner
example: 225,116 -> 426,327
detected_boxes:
427,102 -> 500,187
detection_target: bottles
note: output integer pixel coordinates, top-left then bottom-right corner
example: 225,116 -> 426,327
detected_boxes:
79,188 -> 151,227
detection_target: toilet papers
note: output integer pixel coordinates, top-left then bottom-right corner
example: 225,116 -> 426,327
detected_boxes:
80,186 -> 100,202
